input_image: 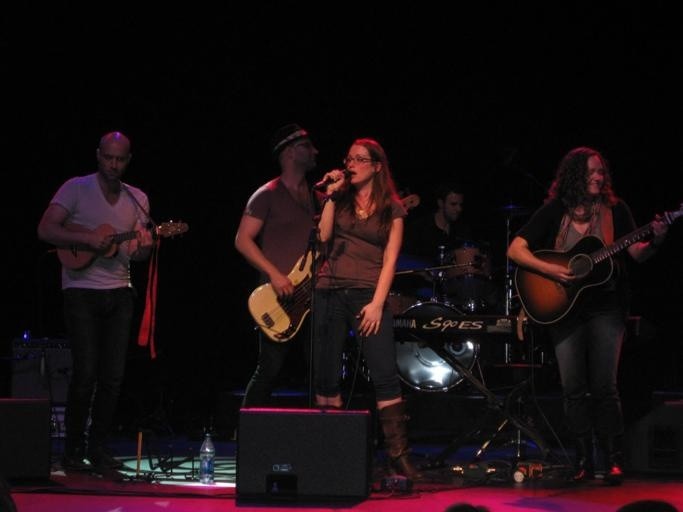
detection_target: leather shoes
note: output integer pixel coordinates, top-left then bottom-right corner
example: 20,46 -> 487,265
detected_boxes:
89,450 -> 127,469
61,444 -> 92,471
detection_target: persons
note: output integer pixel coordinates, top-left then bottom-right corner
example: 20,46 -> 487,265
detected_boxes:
415,187 -> 466,266
231,125 -> 326,408
314,137 -> 424,484
39,131 -> 161,473
508,147 -> 668,487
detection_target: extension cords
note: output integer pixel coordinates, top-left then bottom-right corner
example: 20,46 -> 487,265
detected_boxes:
528,465 -> 543,480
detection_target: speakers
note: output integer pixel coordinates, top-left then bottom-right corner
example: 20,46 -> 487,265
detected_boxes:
1,396 -> 51,490
235,405 -> 374,508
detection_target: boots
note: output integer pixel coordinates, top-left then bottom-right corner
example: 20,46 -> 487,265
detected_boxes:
377,399 -> 425,481
563,430 -> 595,481
601,435 -> 626,485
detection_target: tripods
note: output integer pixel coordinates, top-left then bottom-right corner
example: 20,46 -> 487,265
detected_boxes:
475,314 -> 560,482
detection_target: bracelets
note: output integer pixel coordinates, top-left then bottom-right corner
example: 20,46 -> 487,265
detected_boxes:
322,191 -> 338,202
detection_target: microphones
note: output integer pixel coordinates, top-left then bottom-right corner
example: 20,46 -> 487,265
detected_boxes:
318,168 -> 353,194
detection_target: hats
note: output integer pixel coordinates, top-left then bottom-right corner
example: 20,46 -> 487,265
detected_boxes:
272,125 -> 300,146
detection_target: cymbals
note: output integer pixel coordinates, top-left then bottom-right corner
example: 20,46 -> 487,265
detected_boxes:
501,205 -> 532,215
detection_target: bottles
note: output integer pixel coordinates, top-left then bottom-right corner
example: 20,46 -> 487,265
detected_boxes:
198,433 -> 215,483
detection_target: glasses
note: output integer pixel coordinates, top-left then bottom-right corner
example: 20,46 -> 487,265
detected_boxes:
344,157 -> 379,166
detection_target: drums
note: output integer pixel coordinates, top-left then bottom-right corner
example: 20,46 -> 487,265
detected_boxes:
390,302 -> 479,394
438,241 -> 490,270
446,272 -> 501,315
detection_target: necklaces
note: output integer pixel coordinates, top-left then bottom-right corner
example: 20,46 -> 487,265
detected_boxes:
572,209 -> 593,223
353,193 -> 375,220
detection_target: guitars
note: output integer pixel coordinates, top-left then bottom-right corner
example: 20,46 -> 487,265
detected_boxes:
514,203 -> 683,324
248,194 -> 422,343
55,221 -> 189,271
